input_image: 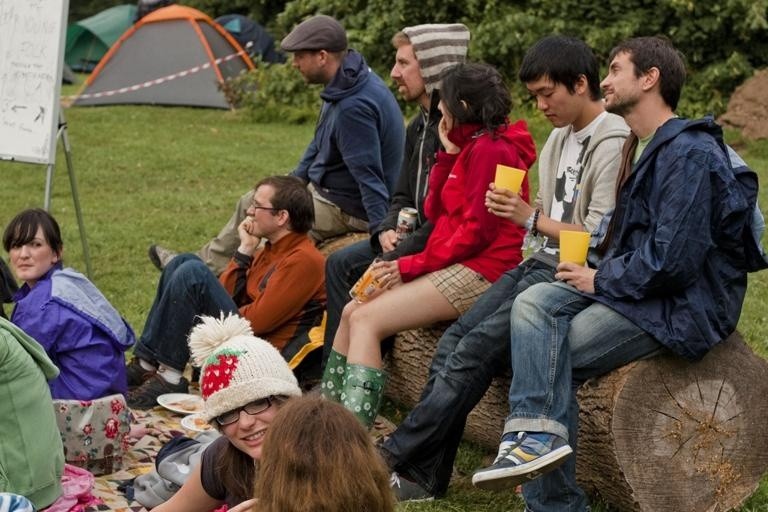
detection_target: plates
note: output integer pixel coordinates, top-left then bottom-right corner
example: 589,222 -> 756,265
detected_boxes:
156,392 -> 204,415
181,415 -> 215,432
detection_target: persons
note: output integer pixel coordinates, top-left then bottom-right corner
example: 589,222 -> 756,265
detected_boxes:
226,396 -> 401,512
321,22 -> 471,369
375,35 -> 632,504
150,312 -> 302,512
124,176 -> 327,410
2,208 -> 136,403
149,15 -> 406,279
0,318 -> 65,511
318,61 -> 537,433
472,37 -> 768,512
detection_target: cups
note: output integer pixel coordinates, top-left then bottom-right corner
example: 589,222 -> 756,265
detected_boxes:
347,258 -> 393,306
559,230 -> 591,267
493,163 -> 526,195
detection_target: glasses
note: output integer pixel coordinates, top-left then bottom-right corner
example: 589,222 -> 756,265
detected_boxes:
216,396 -> 286,427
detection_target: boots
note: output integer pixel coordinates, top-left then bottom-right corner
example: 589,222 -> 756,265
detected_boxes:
320,346 -> 388,434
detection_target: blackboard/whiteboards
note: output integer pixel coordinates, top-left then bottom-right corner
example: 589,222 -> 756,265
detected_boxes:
0,1 -> 71,168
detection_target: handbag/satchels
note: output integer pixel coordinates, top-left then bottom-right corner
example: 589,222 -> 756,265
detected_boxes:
51,394 -> 131,476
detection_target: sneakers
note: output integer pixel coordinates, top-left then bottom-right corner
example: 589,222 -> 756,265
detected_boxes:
390,473 -> 435,505
150,244 -> 178,268
472,431 -> 572,493
123,356 -> 188,410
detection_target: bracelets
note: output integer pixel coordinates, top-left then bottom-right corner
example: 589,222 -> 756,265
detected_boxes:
525,209 -> 542,237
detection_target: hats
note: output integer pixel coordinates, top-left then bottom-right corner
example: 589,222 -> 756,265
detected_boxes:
279,14 -> 348,54
251,202 -> 279,212
186,309 -> 303,424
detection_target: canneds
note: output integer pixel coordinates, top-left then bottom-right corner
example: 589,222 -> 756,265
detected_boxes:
396,207 -> 418,247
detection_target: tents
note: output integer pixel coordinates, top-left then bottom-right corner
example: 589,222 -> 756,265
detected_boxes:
67,2 -> 139,73
65,4 -> 256,110
214,13 -> 287,64
